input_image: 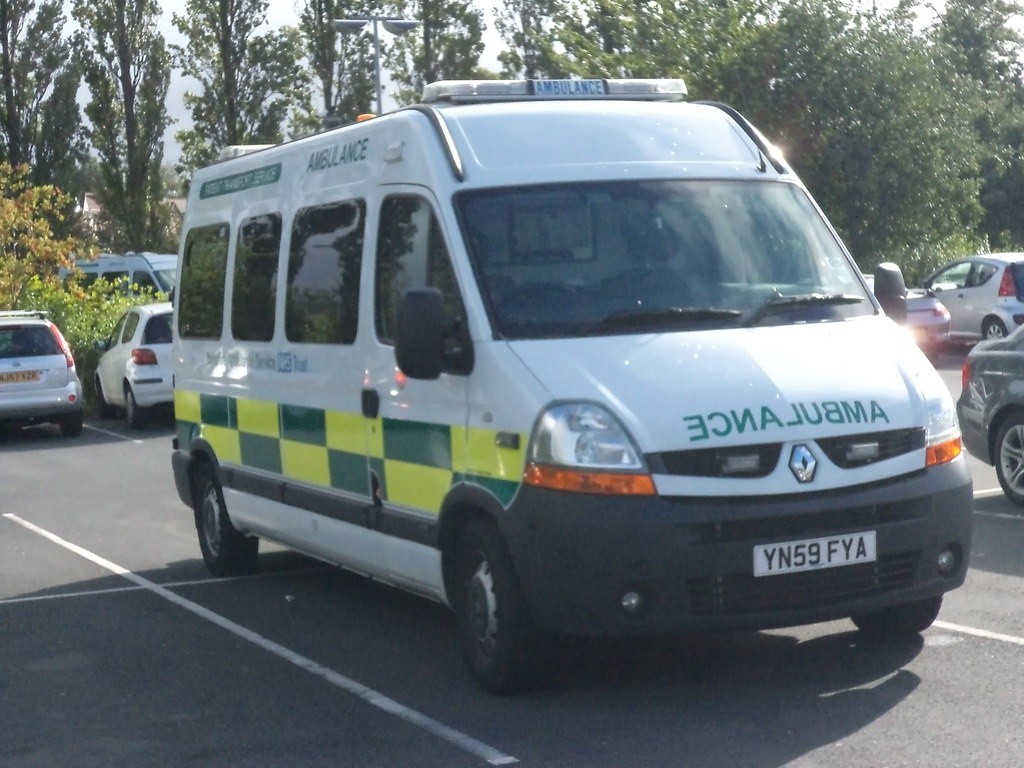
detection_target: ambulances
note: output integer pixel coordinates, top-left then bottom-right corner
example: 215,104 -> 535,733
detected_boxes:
171,79 -> 974,693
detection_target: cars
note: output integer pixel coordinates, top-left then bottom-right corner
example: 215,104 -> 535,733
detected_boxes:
0,311 -> 83,436
957,321 -> 1024,505
94,302 -> 175,428
860,273 -> 951,362
915,253 -> 1024,348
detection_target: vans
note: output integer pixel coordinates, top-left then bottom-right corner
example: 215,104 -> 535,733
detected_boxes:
57,251 -> 178,299
